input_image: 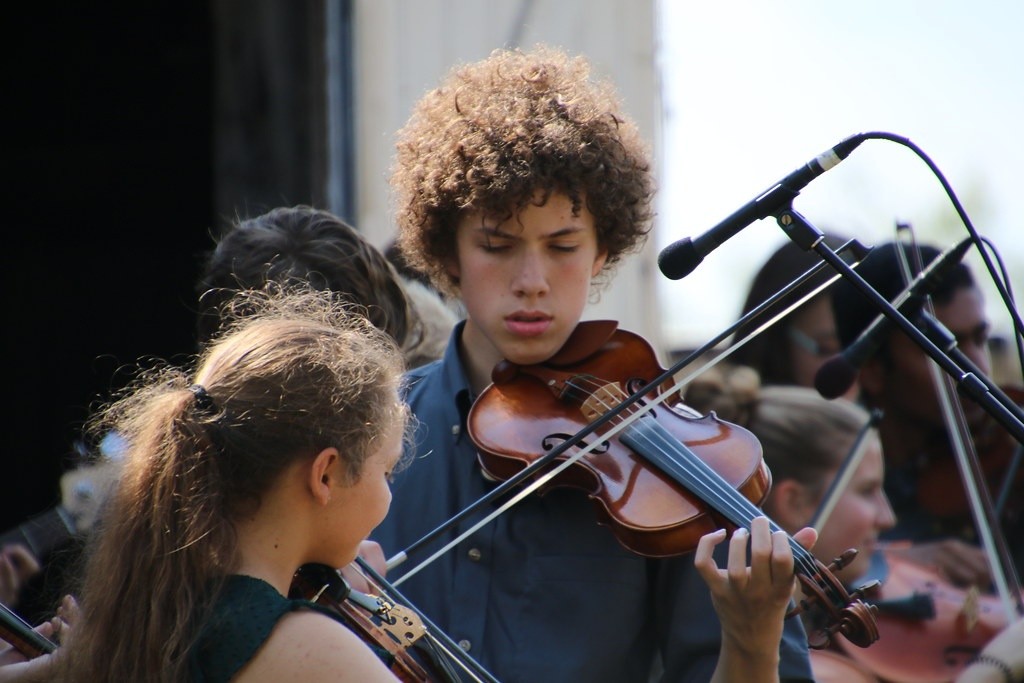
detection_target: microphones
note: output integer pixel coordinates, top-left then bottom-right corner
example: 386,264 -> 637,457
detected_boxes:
815,236 -> 974,400
657,132 -> 867,280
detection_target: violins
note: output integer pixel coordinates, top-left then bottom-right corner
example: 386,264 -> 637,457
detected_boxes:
828,546 -> 1024,683
460,319 -> 886,651
906,383 -> 1024,525
288,563 -> 464,683
0,503 -> 84,661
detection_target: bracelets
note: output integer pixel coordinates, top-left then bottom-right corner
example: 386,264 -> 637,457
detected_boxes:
968,651 -> 1012,681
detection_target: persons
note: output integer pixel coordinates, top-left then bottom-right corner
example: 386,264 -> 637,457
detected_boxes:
374,67 -> 814,683
0,541 -> 91,682
734,233 -> 1023,593
49,281 -> 426,683
687,352 -> 1023,683
196,202 -> 411,365
